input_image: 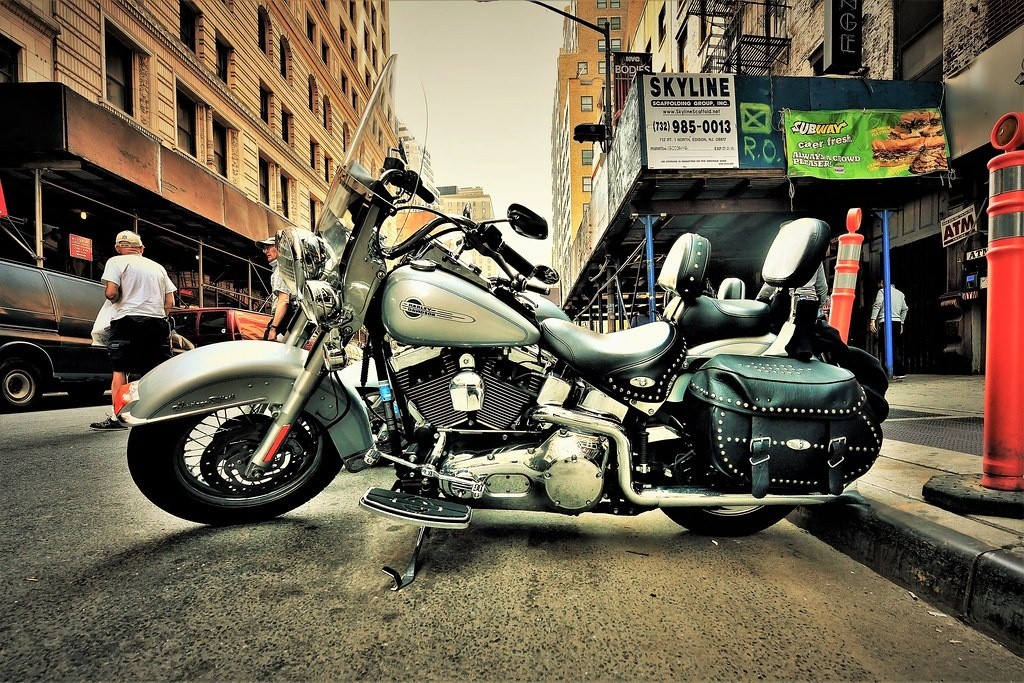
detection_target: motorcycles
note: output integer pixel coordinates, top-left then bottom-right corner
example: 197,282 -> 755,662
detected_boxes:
111,54 -> 892,593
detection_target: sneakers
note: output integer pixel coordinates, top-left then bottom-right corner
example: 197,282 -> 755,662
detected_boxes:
90,412 -> 128,430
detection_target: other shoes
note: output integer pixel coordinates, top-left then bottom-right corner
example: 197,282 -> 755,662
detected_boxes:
893,373 -> 906,379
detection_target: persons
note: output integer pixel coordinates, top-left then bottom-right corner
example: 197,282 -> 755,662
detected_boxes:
255,236 -> 304,341
756,220 -> 829,354
90,230 -> 177,431
870,280 -> 909,379
631,306 -> 649,327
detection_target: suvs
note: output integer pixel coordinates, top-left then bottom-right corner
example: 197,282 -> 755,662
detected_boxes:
168,304 -> 279,354
0,259 -> 196,416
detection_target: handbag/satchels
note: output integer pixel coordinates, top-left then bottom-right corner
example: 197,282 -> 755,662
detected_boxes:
91,298 -> 118,346
683,352 -> 884,497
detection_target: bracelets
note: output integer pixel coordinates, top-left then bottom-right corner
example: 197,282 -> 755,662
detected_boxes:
271,325 -> 278,329
870,322 -> 873,325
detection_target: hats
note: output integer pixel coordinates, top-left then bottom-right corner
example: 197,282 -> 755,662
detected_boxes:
116,230 -> 145,248
255,237 -> 275,249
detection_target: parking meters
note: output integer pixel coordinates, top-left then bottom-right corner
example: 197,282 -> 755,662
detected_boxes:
977,110 -> 1024,490
827,208 -> 866,347
